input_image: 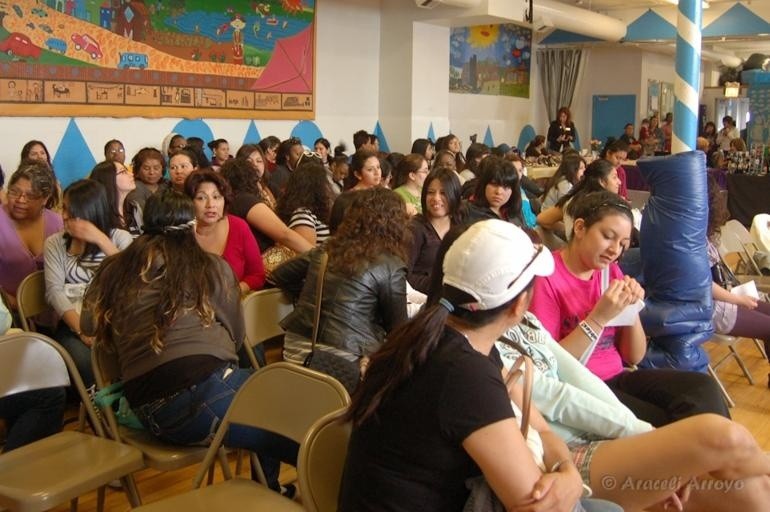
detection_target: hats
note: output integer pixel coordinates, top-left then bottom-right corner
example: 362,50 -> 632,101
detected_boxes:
441,218 -> 556,312
496,144 -> 511,155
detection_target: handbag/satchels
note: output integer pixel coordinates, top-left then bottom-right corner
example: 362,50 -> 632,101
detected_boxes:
303,345 -> 361,397
467,402 -> 551,512
95,382 -> 145,431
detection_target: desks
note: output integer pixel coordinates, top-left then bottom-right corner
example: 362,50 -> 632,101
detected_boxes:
728,172 -> 770,226
621,158 -> 728,195
527,155 -> 600,191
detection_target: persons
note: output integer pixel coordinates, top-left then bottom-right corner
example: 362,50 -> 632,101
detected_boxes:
428,212 -> 770,512
81,188 -> 300,500
0,106 -> 770,428
336,219 -> 623,512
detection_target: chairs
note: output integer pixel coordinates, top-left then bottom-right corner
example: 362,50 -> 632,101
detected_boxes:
0,332 -> 147,511
90,326 -> 268,511
727,220 -> 770,276
127,360 -> 353,512
16,270 -> 97,512
718,225 -> 770,297
702,333 -> 754,407
240,287 -> 297,371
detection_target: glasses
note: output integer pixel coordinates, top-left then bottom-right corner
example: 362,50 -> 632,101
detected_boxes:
171,144 -> 187,149
4,186 -> 45,201
109,148 -> 125,154
